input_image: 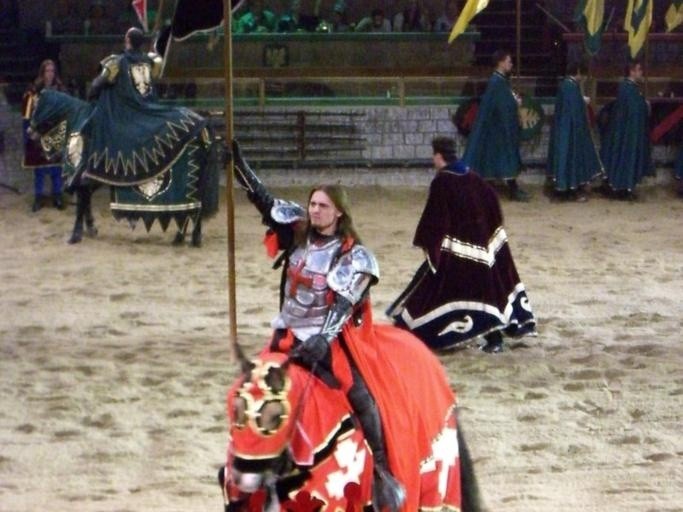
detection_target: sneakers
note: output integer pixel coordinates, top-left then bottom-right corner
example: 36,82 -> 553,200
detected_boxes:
508,188 -> 635,203
480,333 -> 505,353
30,195 -> 68,212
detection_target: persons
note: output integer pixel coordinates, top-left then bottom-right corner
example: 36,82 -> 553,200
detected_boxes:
218,135 -> 408,508
23,58 -> 73,211
601,60 -> 654,200
387,137 -> 539,352
229,0 -> 467,32
463,49 -> 528,202
80,26 -> 209,186
550,61 -> 602,201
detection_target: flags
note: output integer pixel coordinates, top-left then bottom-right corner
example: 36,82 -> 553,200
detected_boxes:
663,0 -> 683,33
573,0 -> 606,56
448,0 -> 492,44
622,0 -> 653,60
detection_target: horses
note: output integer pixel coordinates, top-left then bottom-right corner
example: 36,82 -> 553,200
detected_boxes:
25,84 -> 220,247
218,323 -> 478,512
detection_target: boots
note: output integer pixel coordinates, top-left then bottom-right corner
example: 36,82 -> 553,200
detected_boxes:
358,407 -> 405,507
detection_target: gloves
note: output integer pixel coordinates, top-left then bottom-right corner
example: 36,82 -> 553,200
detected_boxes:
212,135 -> 242,172
297,336 -> 328,364
